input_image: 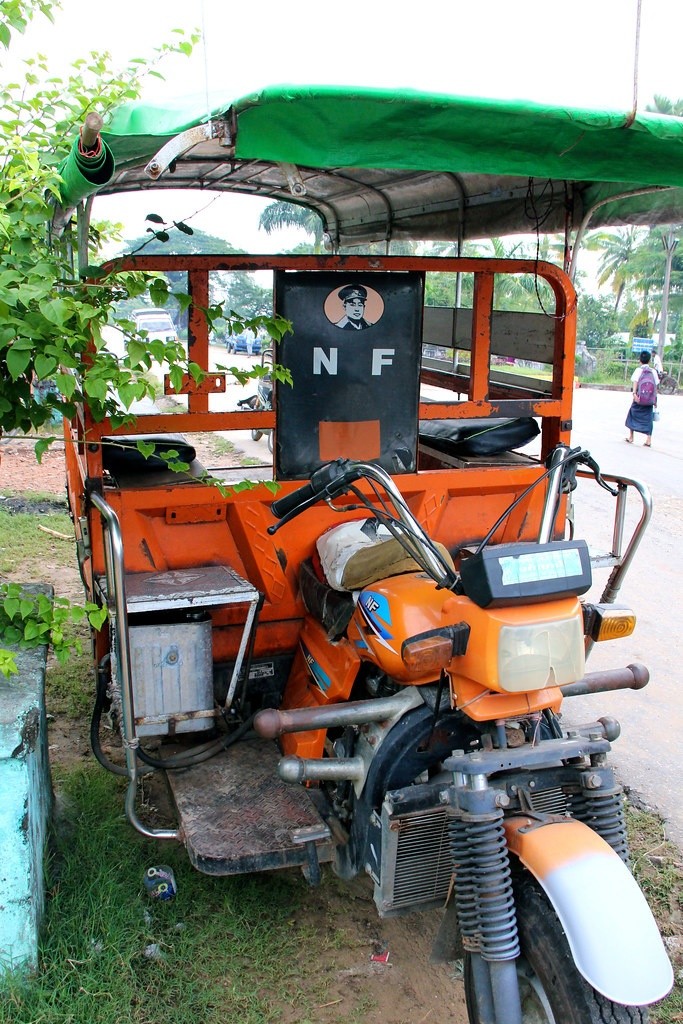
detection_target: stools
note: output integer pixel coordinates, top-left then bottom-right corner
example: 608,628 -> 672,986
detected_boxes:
303,512 -> 459,650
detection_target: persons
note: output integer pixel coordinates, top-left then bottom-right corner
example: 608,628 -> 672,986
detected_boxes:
624,350 -> 659,448
651,350 -> 665,378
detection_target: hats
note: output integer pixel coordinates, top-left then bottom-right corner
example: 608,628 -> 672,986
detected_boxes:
338,285 -> 368,302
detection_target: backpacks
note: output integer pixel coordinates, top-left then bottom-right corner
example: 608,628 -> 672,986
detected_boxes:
638,366 -> 658,406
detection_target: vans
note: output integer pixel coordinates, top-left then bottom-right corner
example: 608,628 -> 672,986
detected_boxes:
124,309 -> 178,356
224,326 -> 262,355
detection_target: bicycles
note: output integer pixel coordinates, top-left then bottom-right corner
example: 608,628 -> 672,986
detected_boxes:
657,372 -> 676,396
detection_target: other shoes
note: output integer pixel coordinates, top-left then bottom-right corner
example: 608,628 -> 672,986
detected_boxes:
625,437 -> 634,443
643,443 -> 652,447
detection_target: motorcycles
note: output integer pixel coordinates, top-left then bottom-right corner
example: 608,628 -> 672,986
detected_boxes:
46,83 -> 683,1023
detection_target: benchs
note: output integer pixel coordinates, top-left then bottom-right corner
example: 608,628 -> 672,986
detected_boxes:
411,387 -> 539,464
81,372 -> 197,480
